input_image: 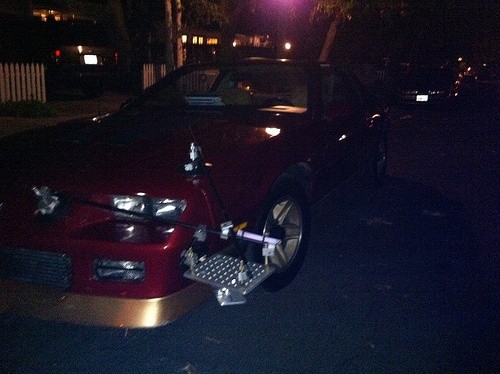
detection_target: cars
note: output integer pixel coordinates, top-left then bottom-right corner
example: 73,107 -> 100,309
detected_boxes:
397,61 -> 465,111
0,57 -> 393,330
37,45 -> 121,102
